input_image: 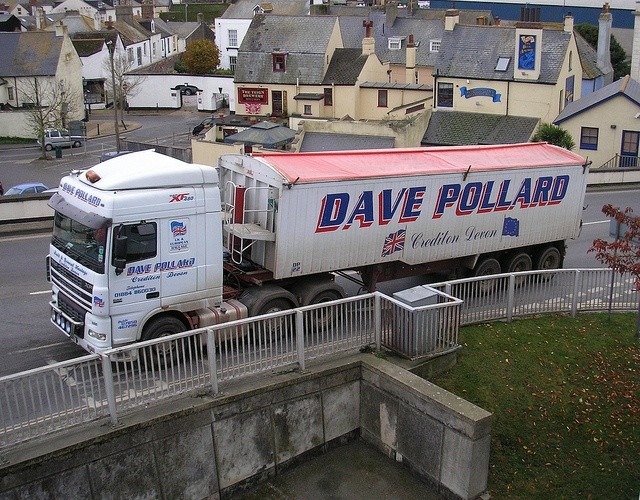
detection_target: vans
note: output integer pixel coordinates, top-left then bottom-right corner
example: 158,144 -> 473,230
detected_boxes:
193,118 -> 220,137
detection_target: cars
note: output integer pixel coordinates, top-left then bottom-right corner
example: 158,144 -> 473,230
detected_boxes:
4,182 -> 48,195
181,87 -> 198,95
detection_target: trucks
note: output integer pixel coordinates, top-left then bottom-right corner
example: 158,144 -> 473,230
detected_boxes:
47,141 -> 592,370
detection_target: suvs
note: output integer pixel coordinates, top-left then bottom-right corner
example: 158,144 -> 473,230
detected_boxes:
37,128 -> 83,151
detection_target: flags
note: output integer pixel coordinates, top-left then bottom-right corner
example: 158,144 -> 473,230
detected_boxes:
171,221 -> 184,232
93,296 -> 103,303
502,217 -> 519,237
96,301 -> 104,308
381,229 -> 406,257
173,227 -> 187,238
